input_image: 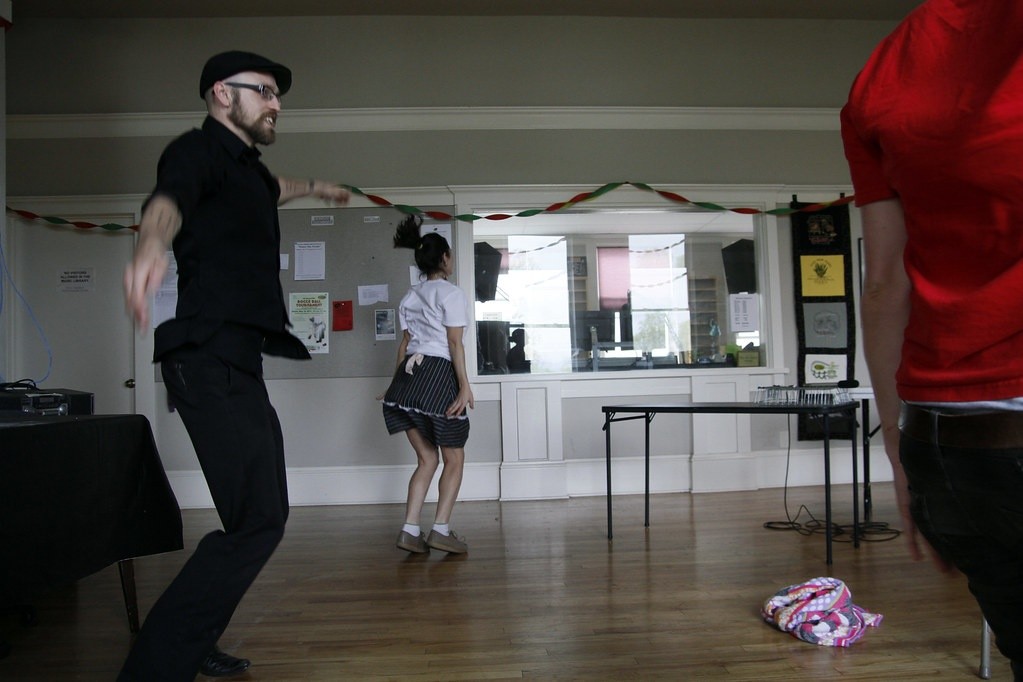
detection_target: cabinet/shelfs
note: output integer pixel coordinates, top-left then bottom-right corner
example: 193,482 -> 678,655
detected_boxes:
686,275 -> 720,349
569,275 -> 587,312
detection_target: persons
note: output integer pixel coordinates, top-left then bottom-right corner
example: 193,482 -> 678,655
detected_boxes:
506,328 -> 532,375
377,212 -> 475,553
120,49 -> 351,682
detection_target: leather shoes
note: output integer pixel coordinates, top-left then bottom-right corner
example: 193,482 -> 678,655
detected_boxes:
200,645 -> 250,677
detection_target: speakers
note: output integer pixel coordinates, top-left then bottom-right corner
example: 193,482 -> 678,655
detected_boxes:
721,238 -> 757,295
473,241 -> 503,303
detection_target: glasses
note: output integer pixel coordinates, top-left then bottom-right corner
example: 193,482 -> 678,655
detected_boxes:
211,82 -> 282,108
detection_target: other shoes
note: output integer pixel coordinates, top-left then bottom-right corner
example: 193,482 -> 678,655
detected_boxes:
426,528 -> 468,552
396,529 -> 427,553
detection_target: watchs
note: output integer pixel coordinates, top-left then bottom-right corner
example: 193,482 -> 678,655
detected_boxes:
839,0 -> 1023,682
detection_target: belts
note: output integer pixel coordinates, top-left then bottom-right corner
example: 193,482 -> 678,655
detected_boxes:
897,399 -> 1023,450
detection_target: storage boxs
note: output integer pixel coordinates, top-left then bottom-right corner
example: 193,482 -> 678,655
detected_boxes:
736,351 -> 761,367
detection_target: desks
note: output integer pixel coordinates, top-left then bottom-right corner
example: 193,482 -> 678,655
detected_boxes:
601,401 -> 861,564
0,411 -> 185,658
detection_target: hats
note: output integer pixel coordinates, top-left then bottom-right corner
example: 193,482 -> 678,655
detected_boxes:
200,51 -> 292,99
